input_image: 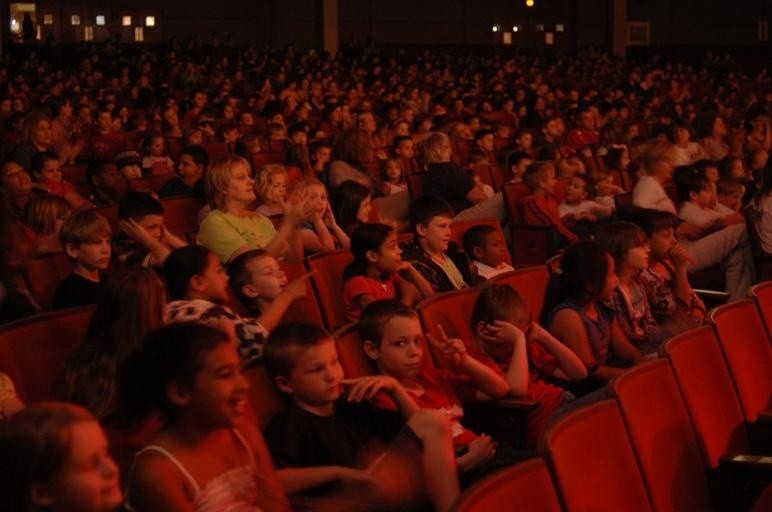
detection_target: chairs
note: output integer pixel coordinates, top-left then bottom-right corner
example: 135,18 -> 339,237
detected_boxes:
2,107 -> 772,511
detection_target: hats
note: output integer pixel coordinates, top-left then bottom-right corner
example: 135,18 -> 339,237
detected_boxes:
116,149 -> 142,170
